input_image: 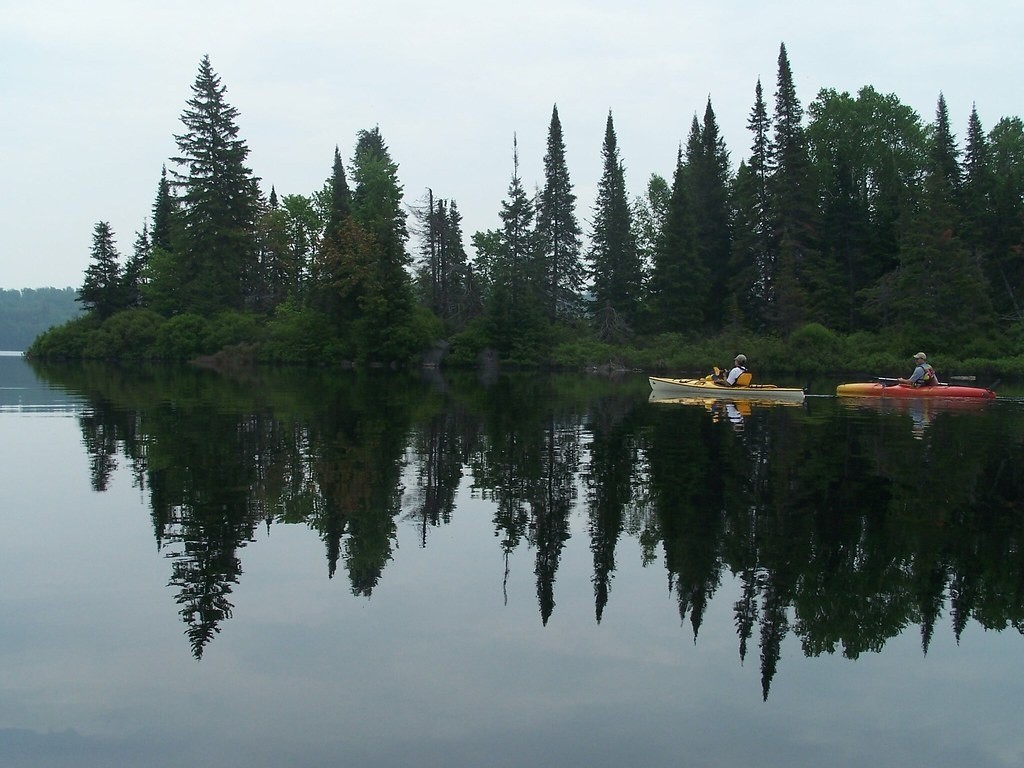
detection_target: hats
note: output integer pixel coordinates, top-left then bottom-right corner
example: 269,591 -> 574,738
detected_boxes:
734,354 -> 746,363
913,352 -> 927,361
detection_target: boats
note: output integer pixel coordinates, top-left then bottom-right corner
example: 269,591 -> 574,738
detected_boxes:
649,376 -> 808,399
836,383 -> 996,400
648,390 -> 803,407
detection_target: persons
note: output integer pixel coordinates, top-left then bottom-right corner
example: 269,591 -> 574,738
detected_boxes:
725,354 -> 748,386
910,406 -> 927,440
724,404 -> 743,432
898,352 -> 938,387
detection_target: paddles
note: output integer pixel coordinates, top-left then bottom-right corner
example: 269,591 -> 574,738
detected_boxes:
713,366 -> 724,376
874,377 -> 964,386
